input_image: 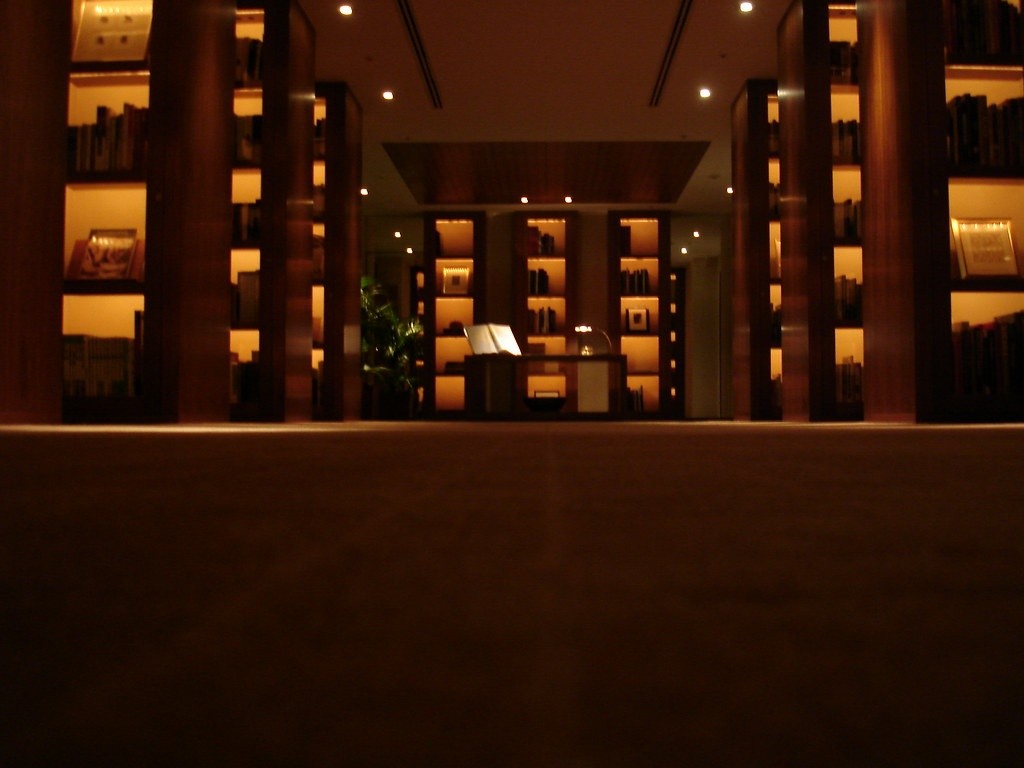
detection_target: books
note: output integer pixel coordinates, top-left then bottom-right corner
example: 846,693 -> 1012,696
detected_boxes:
464,322 -> 522,355
434,226 -> 650,412
61,39 -> 330,412
767,0 -> 1024,411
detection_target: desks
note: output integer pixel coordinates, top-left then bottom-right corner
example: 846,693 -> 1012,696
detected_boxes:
464,354 -> 628,423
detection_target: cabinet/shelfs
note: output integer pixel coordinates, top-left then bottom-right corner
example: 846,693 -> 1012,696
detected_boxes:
0,0 -> 1024,424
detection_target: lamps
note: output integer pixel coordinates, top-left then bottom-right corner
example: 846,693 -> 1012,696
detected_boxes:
574,323 -> 615,353
